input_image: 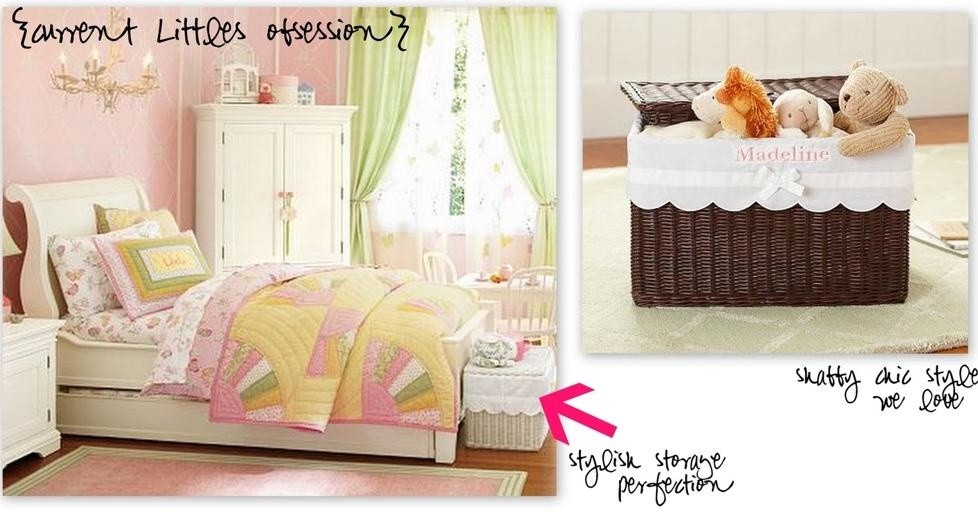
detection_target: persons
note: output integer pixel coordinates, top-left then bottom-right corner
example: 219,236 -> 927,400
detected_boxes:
832,61 -> 908,156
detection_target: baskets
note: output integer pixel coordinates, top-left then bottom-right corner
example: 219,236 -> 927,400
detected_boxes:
627,111 -> 911,308
463,346 -> 553,451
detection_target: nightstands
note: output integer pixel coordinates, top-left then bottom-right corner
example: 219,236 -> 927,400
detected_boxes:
4,316 -> 67,468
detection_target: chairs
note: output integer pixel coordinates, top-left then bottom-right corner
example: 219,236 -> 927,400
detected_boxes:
421,252 -> 457,285
499,266 -> 555,346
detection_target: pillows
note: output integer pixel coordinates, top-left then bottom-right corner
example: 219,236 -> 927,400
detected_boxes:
47,220 -> 167,318
92,204 -> 182,238
88,227 -> 218,320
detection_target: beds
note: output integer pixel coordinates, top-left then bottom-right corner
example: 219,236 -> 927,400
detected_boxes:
5,173 -> 499,470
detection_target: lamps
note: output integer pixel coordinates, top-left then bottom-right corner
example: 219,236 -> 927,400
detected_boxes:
41,5 -> 169,116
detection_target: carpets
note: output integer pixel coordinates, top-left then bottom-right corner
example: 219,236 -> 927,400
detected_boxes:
2,444 -> 530,496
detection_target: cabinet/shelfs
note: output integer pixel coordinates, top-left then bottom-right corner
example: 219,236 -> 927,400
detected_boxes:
185,104 -> 359,278
